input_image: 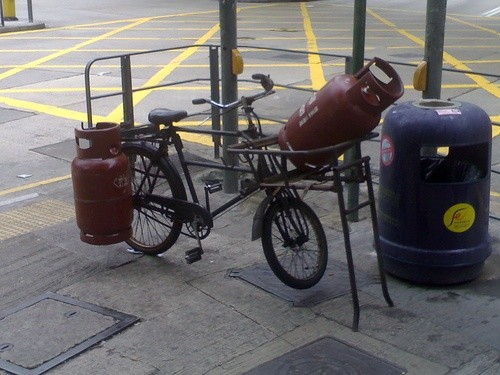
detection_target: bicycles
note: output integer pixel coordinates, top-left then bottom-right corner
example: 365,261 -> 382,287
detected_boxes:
120,72 -> 395,331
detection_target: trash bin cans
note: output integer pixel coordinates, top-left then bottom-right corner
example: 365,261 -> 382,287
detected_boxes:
374,99 -> 492,287
